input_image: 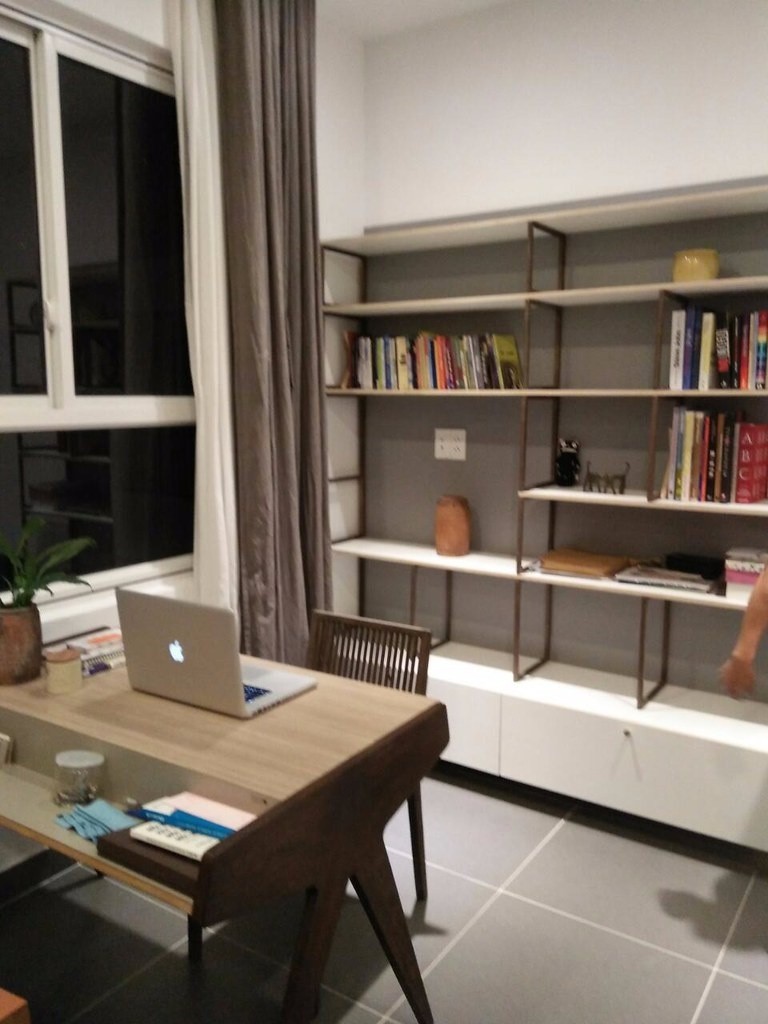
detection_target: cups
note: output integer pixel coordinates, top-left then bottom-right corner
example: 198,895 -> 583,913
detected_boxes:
53,751 -> 104,805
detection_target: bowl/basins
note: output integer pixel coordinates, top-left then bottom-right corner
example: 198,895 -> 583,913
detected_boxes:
672,249 -> 720,281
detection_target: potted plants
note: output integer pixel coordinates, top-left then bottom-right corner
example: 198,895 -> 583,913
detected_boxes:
0,515 -> 96,686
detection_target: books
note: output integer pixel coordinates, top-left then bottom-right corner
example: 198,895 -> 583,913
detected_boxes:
540,548 -> 720,593
724,546 -> 768,605
355,332 -> 522,391
668,309 -> 768,390
660,405 -> 768,503
97,790 -> 256,897
41,627 -> 126,676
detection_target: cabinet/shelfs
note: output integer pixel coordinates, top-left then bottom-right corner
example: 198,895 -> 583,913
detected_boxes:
325,173 -> 768,853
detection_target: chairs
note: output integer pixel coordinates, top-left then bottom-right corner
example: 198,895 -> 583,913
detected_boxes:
190,608 -> 433,970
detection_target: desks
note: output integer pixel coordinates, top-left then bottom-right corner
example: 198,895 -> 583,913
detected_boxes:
0,642 -> 451,1024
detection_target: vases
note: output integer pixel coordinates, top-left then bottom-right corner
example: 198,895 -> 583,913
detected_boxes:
673,248 -> 720,282
436,496 -> 472,555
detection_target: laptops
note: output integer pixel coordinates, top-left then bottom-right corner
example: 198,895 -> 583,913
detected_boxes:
115,588 -> 319,720
